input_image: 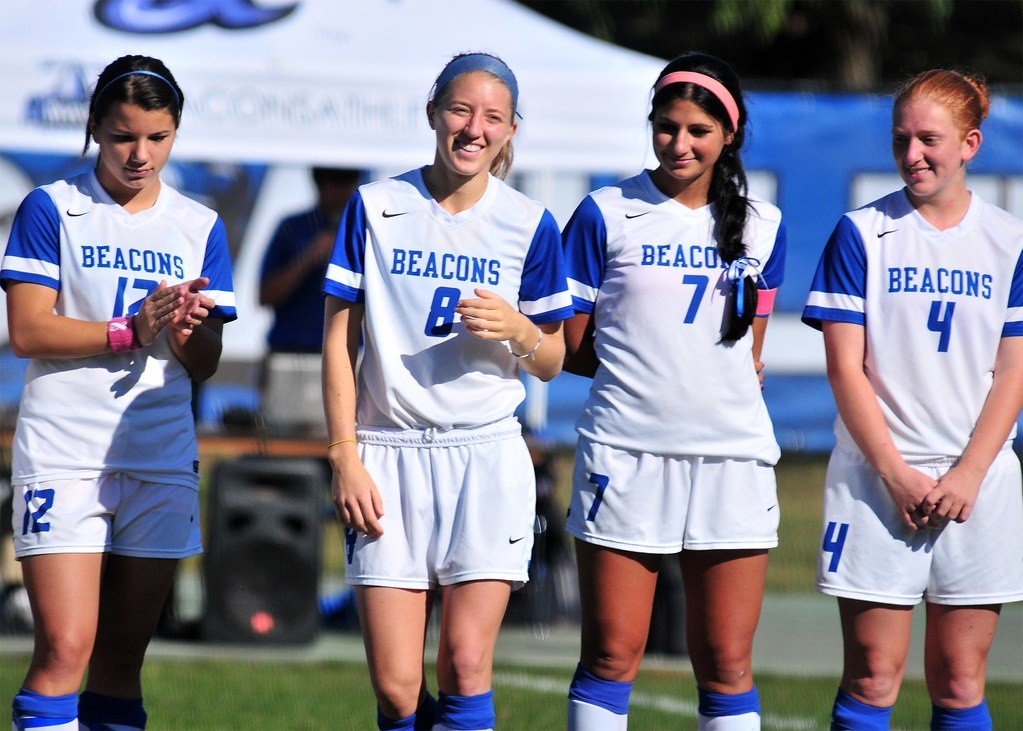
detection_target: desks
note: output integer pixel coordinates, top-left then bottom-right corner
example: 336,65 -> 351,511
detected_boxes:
193,423 -> 547,469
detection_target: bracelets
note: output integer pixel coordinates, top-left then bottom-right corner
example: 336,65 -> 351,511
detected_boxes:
511,328 -> 542,361
107,317 -> 142,354
325,439 -> 356,450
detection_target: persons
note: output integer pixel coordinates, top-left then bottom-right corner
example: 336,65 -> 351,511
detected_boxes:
800,68 -> 1023,731
548,52 -> 782,731
1,57 -> 237,731
316,51 -> 575,731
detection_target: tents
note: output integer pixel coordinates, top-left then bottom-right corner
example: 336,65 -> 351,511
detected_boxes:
0,0 -> 1023,434
